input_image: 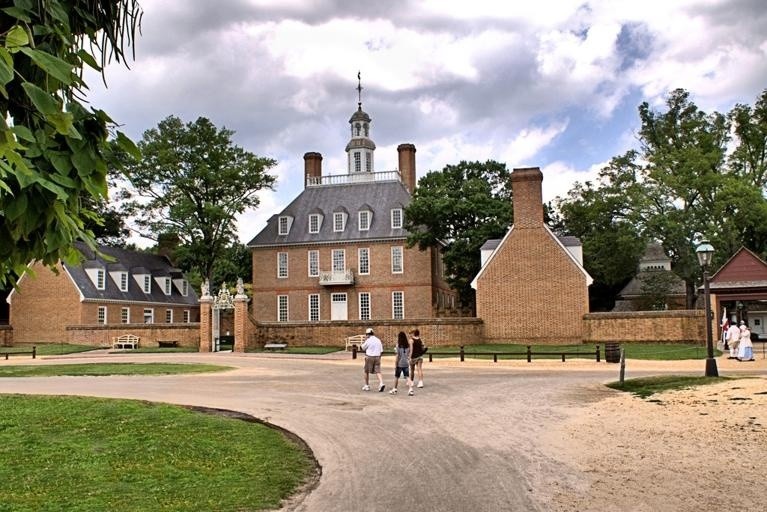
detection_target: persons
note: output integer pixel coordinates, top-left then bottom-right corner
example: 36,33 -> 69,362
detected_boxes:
389,332 -> 414,396
360,328 -> 385,392
406,329 -> 424,388
726,320 -> 755,361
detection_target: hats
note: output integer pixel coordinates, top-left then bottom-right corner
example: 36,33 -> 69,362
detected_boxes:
740,325 -> 746,330
366,328 -> 374,333
730,321 -> 737,325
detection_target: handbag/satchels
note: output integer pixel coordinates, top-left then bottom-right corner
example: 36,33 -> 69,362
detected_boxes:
422,345 -> 428,353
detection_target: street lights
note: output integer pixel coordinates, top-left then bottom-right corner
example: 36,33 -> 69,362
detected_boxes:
696,240 -> 719,377
737,303 -> 744,320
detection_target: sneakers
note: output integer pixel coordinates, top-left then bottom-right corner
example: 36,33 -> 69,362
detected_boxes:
728,356 -> 755,361
362,381 -> 423,395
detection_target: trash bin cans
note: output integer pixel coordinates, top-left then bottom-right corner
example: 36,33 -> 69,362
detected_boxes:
605,343 -> 620,363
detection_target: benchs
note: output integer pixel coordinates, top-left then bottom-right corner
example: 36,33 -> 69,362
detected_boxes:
111,333 -> 140,351
153,338 -> 178,348
344,334 -> 369,352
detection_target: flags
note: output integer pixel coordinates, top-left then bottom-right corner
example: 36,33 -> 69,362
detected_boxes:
720,307 -> 730,345
